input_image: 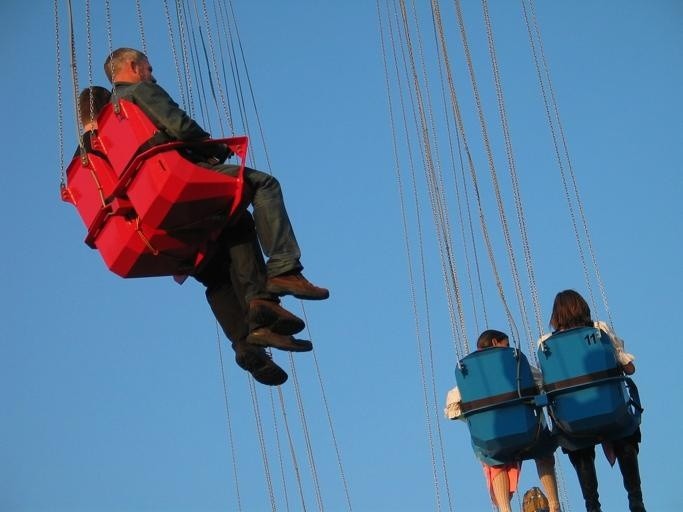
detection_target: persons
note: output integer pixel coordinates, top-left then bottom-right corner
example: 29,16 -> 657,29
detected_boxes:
537,294 -> 650,511
444,330 -> 561,512
62,86 -> 313,386
102,47 -> 328,333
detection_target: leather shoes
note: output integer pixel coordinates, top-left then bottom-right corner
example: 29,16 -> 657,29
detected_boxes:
233,343 -> 288,386
248,299 -> 305,336
265,271 -> 329,300
247,326 -> 312,352
